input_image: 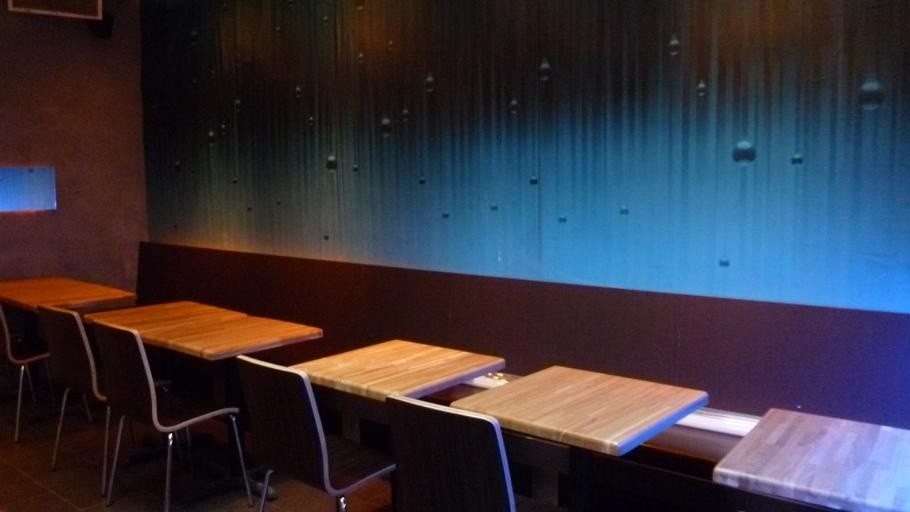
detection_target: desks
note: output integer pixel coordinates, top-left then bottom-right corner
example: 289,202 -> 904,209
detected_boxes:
82,299 -> 323,499
0,276 -> 139,318
709,407 -> 909,512
445,363 -> 709,512
288,339 -> 506,512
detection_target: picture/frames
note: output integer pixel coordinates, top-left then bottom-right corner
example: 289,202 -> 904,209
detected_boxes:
8,1 -> 103,20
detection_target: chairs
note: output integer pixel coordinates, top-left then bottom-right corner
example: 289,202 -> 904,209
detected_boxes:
236,353 -> 397,512
90,319 -> 253,512
39,305 -> 173,498
0,306 -> 54,444
384,392 -> 517,512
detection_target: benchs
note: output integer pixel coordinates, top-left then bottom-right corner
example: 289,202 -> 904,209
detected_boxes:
134,242 -> 910,509
